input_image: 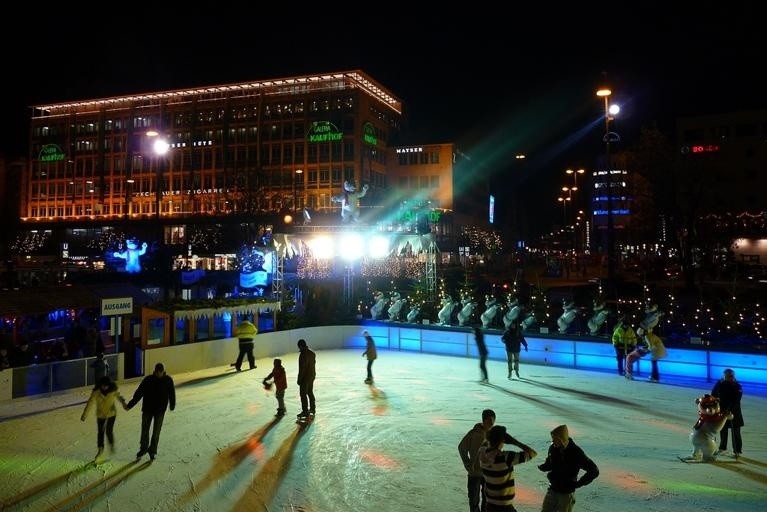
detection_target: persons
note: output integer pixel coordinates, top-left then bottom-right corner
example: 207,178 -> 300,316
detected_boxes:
361,330 -> 376,383
472,328 -> 489,382
502,320 -> 528,378
125,364 -> 175,460
712,369 -> 744,457
478,426 -> 538,512
81,376 -> 128,462
537,425 -> 599,512
612,320 -> 667,384
263,359 -> 287,415
297,339 -> 315,417
235,316 -> 258,371
0,319 -> 110,389
458,410 -> 495,512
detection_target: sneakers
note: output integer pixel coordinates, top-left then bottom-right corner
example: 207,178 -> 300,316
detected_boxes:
137,450 -> 156,460
364,378 -> 375,384
94,443 -> 116,459
619,370 -> 659,383
273,408 -> 316,418
235,363 -> 258,371
507,370 -> 520,378
692,447 -> 743,462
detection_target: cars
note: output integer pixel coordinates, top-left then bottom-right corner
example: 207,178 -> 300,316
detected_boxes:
488,276 -> 538,297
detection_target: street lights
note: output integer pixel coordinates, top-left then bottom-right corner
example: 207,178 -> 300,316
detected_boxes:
293,168 -> 304,225
555,168 -> 585,280
147,123 -> 167,218
596,88 -> 621,300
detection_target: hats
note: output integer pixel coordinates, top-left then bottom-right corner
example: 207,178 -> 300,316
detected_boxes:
361,330 -> 369,336
297,339 -> 306,346
155,363 -> 165,372
